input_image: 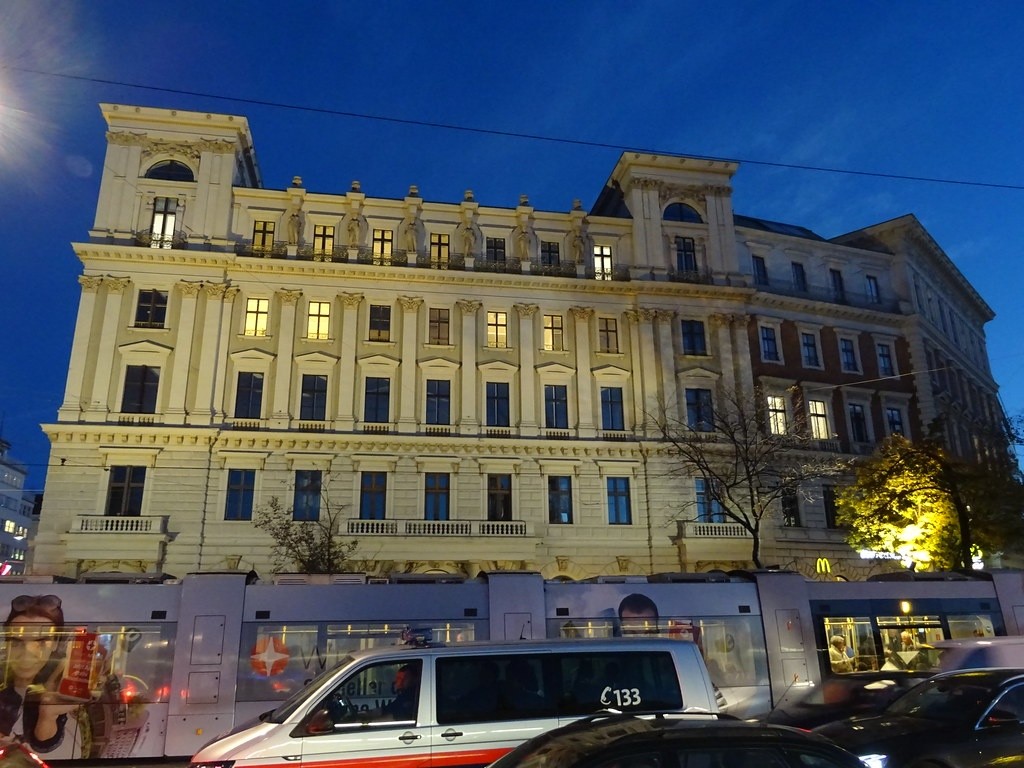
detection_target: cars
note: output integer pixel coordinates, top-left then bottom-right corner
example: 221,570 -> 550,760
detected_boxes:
478,635 -> 1024,768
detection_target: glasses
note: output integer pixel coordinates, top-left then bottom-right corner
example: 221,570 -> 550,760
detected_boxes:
11,594 -> 62,612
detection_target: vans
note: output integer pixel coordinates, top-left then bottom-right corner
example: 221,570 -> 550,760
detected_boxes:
188,633 -> 724,768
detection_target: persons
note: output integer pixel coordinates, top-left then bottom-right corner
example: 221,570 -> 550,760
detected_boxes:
358,665 -> 416,721
829,629 -> 922,673
618,593 -> 728,715
406,218 -> 417,253
517,226 -> 531,261
288,211 -> 301,245
462,222 -> 475,258
572,230 -> 585,264
347,213 -> 361,249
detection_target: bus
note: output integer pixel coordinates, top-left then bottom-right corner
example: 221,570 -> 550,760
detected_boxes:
0,570 -> 1024,767
